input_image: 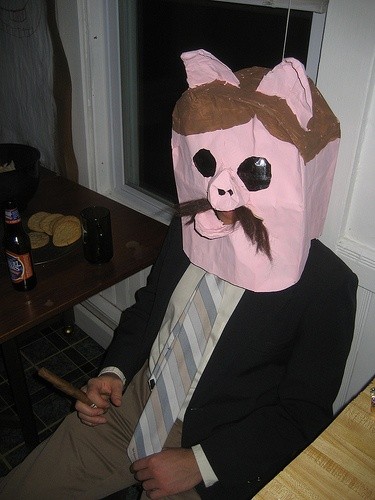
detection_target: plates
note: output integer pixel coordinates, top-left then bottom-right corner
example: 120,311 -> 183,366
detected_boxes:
24,210 -> 84,264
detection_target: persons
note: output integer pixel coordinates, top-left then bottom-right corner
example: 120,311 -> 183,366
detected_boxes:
0,0 -> 79,182
0,49 -> 358,500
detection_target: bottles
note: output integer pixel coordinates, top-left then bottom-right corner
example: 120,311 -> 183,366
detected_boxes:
5,198 -> 37,290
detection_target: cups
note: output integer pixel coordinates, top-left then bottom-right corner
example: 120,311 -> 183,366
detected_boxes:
80,207 -> 113,262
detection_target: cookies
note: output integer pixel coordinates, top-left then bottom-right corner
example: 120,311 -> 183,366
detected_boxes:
25,212 -> 83,249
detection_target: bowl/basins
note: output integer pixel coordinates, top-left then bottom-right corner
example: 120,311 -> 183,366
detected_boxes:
0,143 -> 40,197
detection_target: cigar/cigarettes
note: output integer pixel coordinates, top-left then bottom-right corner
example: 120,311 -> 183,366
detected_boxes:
38,367 -> 92,406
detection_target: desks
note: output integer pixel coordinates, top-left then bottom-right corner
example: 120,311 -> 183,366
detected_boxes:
0,166 -> 169,447
252,376 -> 375,500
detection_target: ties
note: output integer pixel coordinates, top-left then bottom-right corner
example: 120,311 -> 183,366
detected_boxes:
127,271 -> 226,466
